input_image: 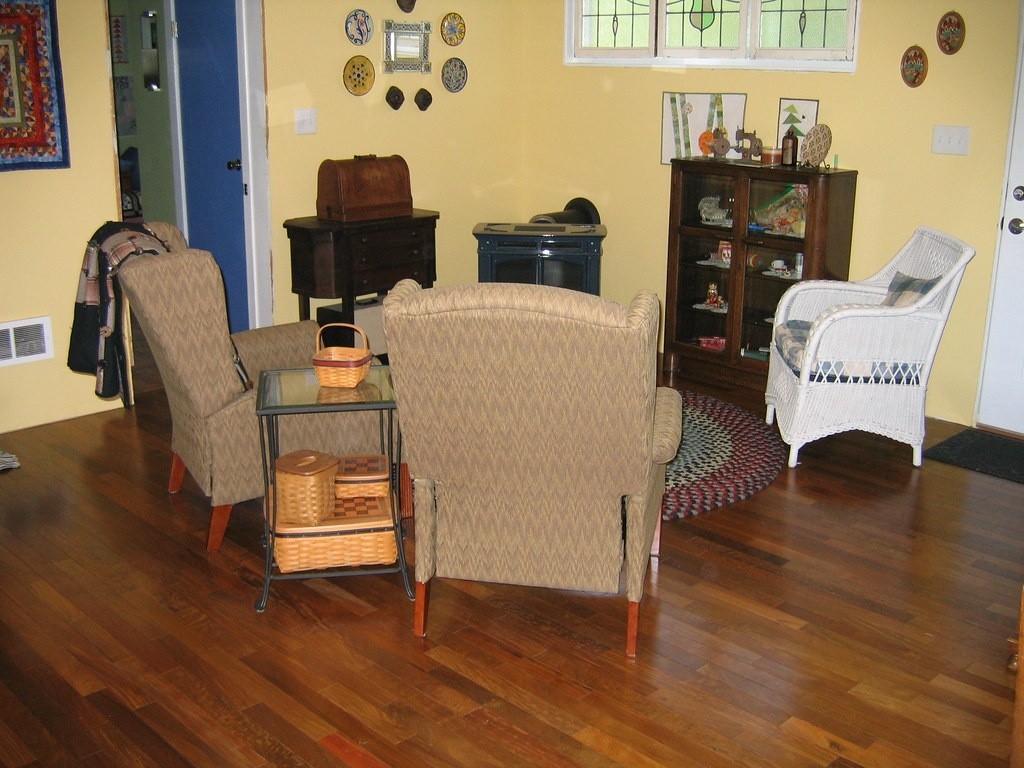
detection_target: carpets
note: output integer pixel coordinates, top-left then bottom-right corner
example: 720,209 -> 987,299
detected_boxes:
661,383 -> 787,521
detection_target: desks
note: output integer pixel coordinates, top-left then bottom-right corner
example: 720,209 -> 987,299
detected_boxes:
282,209 -> 440,364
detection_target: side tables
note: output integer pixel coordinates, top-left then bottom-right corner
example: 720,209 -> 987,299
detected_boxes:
253,363 -> 416,615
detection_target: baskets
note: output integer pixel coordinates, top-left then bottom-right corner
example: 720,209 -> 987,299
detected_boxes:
312,323 -> 374,388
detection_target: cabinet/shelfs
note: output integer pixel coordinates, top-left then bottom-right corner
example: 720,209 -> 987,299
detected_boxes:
472,222 -> 607,296
662,156 -> 858,394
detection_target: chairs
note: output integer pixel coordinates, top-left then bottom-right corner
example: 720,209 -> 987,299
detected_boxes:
66,220 -> 413,555
382,278 -> 683,659
764,224 -> 977,469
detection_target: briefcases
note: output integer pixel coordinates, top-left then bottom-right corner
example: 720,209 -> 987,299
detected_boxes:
316,155 -> 415,221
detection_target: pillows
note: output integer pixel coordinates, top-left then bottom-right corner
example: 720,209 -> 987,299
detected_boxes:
878,271 -> 943,307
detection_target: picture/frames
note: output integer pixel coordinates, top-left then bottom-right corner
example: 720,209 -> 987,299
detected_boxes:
776,97 -> 820,164
659,91 -> 747,166
381,18 -> 433,74
936,11 -> 966,55
901,45 -> 929,89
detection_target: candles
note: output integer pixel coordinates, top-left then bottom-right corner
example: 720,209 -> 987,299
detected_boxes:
760,145 -> 782,165
